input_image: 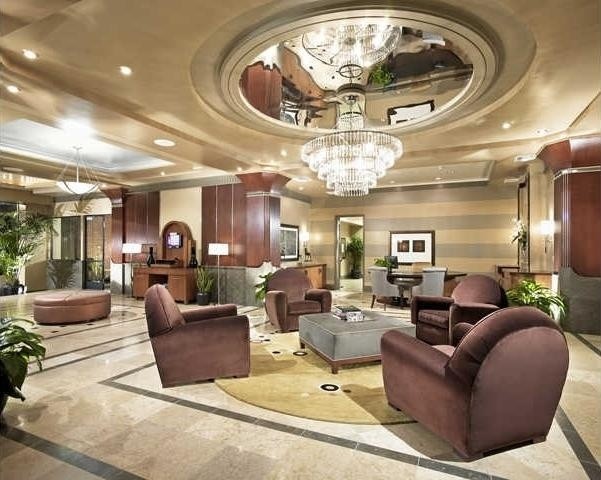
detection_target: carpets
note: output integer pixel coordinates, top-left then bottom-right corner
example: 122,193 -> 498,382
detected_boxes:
214,323 -> 419,425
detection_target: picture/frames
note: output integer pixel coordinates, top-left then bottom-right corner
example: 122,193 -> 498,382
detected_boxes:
389,230 -> 435,266
279,223 -> 299,261
341,238 -> 346,259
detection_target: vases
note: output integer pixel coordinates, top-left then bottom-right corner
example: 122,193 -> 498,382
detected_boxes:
189,246 -> 198,268
147,247 -> 155,268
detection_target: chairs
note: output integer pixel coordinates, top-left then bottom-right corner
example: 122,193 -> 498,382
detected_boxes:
411,275 -> 507,344
379,305 -> 569,463
144,283 -> 250,388
408,267 -> 446,309
266,267 -> 332,333
366,267 -> 403,311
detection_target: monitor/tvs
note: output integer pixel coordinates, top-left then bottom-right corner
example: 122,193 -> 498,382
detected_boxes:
166,231 -> 181,248
384,256 -> 398,268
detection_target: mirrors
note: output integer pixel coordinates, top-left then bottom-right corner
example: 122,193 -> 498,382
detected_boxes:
217,12 -> 502,141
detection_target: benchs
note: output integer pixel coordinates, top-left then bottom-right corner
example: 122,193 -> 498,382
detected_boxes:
32,288 -> 111,325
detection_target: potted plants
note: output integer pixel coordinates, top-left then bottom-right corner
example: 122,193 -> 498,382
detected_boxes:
0,317 -> 46,422
194,266 -> 216,305
509,217 -> 527,254
346,235 -> 364,279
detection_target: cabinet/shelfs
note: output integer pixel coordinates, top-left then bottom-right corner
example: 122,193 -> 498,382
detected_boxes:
132,267 -> 196,305
286,263 -> 326,289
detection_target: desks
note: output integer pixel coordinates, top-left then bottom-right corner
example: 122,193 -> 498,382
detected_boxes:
389,272 -> 467,307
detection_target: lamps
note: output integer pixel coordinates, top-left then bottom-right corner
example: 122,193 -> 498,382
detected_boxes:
299,232 -> 310,249
302,23 -> 402,107
208,242 -> 229,305
55,145 -> 100,195
121,243 -> 141,298
540,220 -> 553,254
301,112 -> 403,199
